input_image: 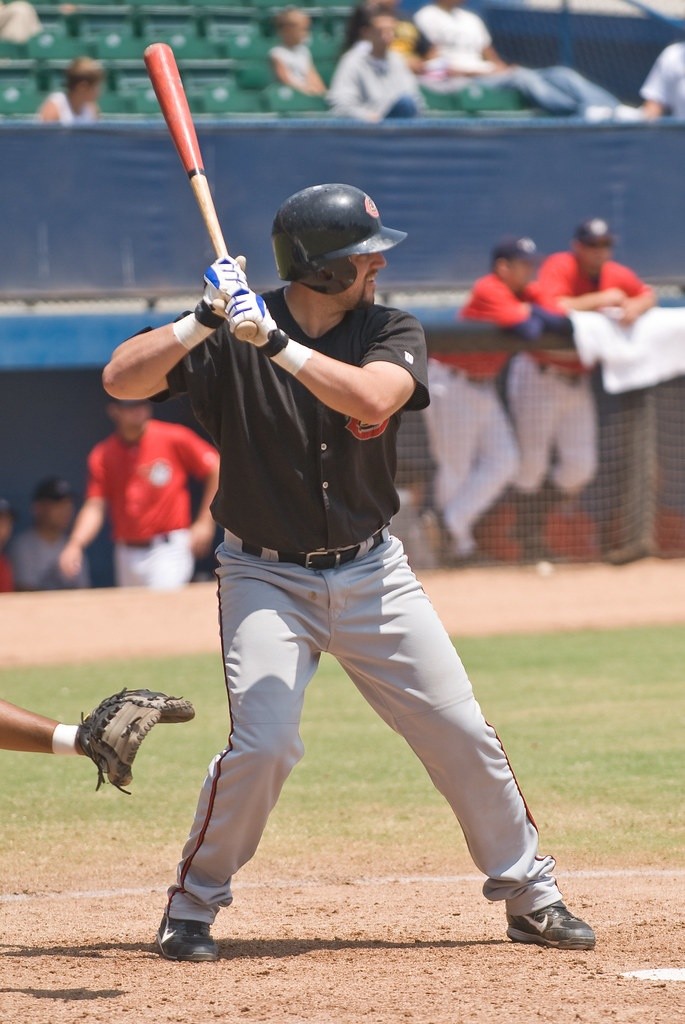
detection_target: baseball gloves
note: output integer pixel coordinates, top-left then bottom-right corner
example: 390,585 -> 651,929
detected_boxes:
78,686 -> 196,798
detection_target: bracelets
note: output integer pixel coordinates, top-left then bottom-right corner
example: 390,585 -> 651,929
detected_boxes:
52,724 -> 80,756
271,339 -> 311,375
174,313 -> 215,352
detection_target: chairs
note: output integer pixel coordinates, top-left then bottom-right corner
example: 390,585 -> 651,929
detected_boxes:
1,0 -> 555,120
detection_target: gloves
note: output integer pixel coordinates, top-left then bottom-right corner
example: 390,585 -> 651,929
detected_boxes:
173,255 -> 248,350
224,288 -> 314,377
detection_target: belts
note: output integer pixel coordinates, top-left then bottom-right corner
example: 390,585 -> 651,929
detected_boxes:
243,530 -> 385,571
125,534 -> 169,549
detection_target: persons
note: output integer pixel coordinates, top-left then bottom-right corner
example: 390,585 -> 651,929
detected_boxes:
101,183 -> 594,960
60,399 -> 220,585
326,0 -> 426,119
0,476 -> 94,592
409,233 -> 572,568
637,40 -> 685,120
37,56 -> 104,121
411,0 -> 643,123
269,8 -> 328,99
0,0 -> 41,40
0,687 -> 195,787
505,219 -> 653,571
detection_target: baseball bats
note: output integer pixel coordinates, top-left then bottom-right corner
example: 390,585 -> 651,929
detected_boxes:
141,41 -> 260,343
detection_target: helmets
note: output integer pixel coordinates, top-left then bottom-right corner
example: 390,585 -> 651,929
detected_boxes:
272,183 -> 408,296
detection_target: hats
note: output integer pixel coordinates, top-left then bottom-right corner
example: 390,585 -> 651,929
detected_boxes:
575,216 -> 616,248
491,236 -> 545,262
34,477 -> 74,498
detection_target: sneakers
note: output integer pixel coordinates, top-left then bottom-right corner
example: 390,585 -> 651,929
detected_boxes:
508,900 -> 594,949
156,914 -> 219,961
